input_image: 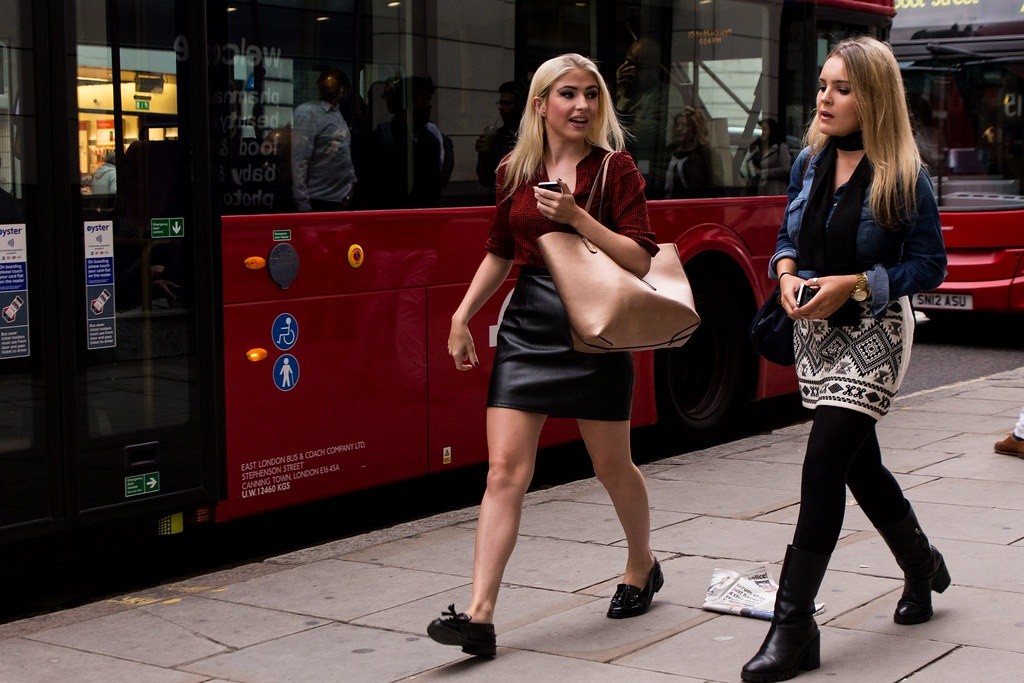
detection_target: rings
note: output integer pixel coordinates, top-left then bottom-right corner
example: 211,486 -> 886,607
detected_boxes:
813,278 -> 818,285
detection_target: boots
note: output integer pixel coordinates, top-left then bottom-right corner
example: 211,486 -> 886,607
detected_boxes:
873,496 -> 952,622
740,543 -> 831,680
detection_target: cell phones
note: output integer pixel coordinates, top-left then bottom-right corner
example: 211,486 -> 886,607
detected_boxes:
538,181 -> 561,194
796,284 -> 816,308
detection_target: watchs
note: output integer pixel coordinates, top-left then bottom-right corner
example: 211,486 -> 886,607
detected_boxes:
851,273 -> 868,302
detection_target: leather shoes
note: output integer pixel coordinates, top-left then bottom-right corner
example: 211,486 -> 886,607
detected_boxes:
428,604 -> 497,657
993,432 -> 1024,458
607,557 -> 664,618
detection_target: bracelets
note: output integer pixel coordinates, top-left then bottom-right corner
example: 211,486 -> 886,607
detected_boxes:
778,272 -> 793,284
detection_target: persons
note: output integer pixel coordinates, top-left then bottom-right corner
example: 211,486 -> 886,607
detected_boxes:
616,37 -> 687,201
90,152 -> 116,194
230,128 -> 310,215
291,68 -> 528,212
993,406 -> 1024,459
426,53 -> 665,656
663,106 -> 724,200
741,36 -> 951,683
739,118 -> 792,196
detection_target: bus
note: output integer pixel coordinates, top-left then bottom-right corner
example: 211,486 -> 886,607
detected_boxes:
797,0 -> 1024,324
0,0 -> 902,552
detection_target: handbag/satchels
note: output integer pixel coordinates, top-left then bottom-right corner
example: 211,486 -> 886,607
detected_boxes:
751,151 -> 818,367
537,152 -> 702,352
